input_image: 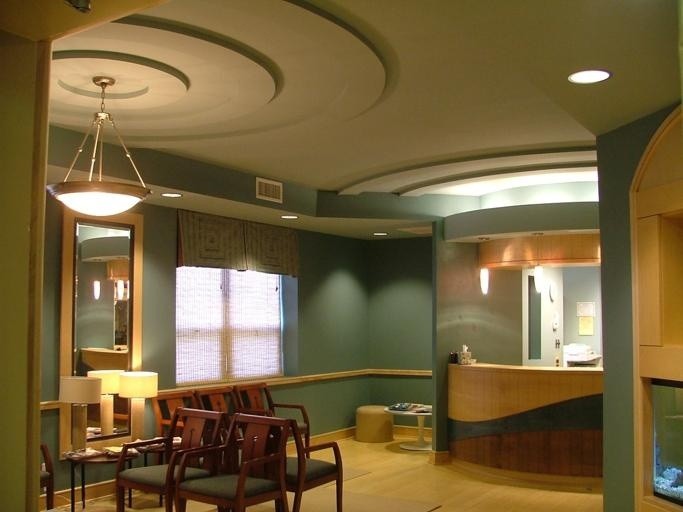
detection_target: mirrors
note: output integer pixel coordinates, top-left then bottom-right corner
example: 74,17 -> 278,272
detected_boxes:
58,203 -> 148,460
518,265 -> 602,367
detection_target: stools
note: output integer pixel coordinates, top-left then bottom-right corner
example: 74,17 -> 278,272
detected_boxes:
354,404 -> 393,443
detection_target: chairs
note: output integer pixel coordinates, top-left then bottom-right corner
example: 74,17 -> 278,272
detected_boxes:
113,382 -> 347,510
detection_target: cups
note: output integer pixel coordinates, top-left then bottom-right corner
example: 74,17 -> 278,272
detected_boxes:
449,352 -> 458,364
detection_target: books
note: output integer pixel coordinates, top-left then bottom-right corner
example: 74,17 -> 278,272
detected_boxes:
389,403 -> 433,413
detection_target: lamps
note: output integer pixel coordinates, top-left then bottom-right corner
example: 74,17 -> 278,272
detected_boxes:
117,369 -> 160,444
87,368 -> 125,436
58,374 -> 102,461
48,70 -> 148,219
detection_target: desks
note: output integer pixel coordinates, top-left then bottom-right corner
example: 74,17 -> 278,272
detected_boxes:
385,405 -> 433,451
